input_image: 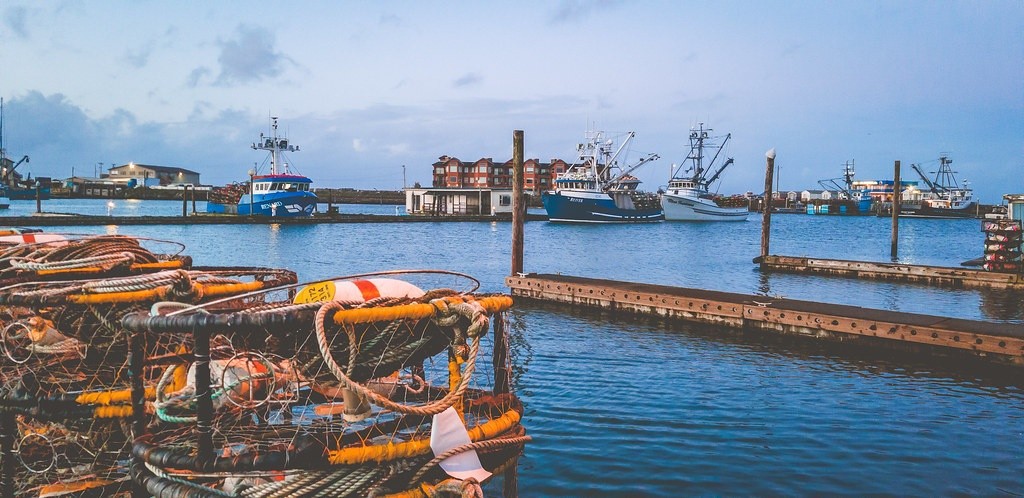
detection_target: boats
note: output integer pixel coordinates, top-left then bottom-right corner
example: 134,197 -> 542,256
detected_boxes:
661,121 -> 750,221
884,149 -> 973,218
548,129 -> 666,224
800,159 -> 872,214
204,113 -> 319,216
